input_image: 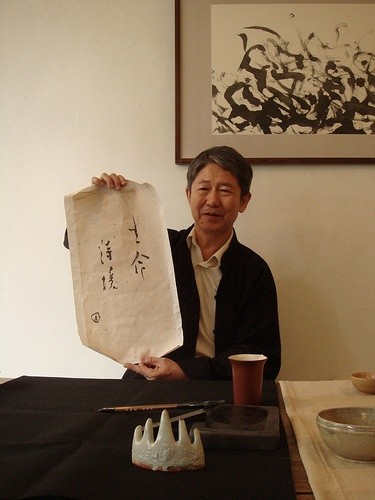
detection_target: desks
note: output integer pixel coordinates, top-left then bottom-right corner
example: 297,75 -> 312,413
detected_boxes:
1,376 -> 374,500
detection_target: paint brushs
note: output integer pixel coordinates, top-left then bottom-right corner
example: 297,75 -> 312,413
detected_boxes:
93,400 -> 223,412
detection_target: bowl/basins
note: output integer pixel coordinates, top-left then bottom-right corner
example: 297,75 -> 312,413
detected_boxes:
349,372 -> 375,394
315,406 -> 375,464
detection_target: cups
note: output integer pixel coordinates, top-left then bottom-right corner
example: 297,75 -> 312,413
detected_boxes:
226,352 -> 267,403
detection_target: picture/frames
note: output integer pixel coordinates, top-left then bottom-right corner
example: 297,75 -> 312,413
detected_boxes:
173,0 -> 375,164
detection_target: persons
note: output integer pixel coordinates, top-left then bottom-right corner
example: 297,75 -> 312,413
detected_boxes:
64,146 -> 283,380
